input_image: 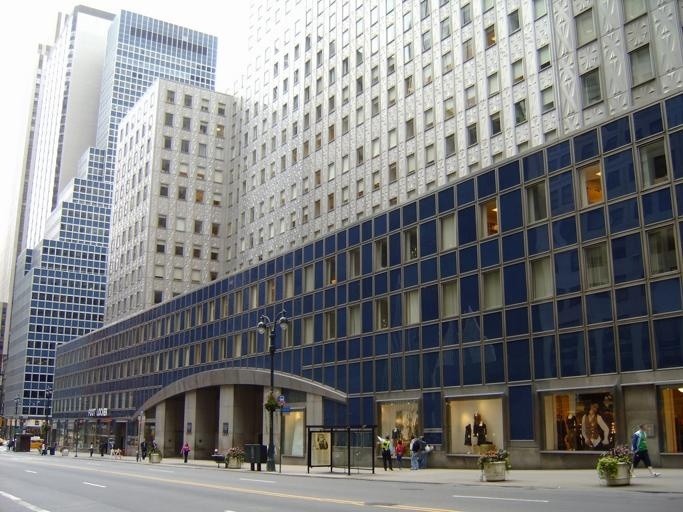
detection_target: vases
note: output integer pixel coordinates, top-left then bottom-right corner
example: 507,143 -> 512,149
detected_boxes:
228,457 -> 240,469
150,453 -> 160,464
606,462 -> 633,486
483,461 -> 506,481
61,449 -> 68,456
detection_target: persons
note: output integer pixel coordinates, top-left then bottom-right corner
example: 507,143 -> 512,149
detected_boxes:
630,424 -> 662,478
376,433 -> 393,470
395,440 -> 404,469
476,421 -> 487,445
582,401 -> 610,451
473,409 -> 481,436
41,442 -> 47,456
564,411 -> 579,450
141,438 -> 147,460
410,435 -> 434,470
181,443 -> 191,463
391,426 -> 400,449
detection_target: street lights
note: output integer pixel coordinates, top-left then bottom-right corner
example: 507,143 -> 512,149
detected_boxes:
13,394 -> 20,451
256,307 -> 289,472
43,385 -> 53,445
73,418 -> 83,458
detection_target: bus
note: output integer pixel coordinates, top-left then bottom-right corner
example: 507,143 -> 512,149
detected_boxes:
29,439 -> 44,449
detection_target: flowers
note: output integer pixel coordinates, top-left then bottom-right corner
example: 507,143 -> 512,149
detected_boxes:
263,395 -> 288,411
594,446 -> 634,480
474,449 -> 509,482
224,448 -> 244,468
59,445 -> 69,453
146,439 -> 163,463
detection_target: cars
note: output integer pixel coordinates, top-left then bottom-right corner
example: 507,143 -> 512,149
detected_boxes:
2,438 -> 16,447
